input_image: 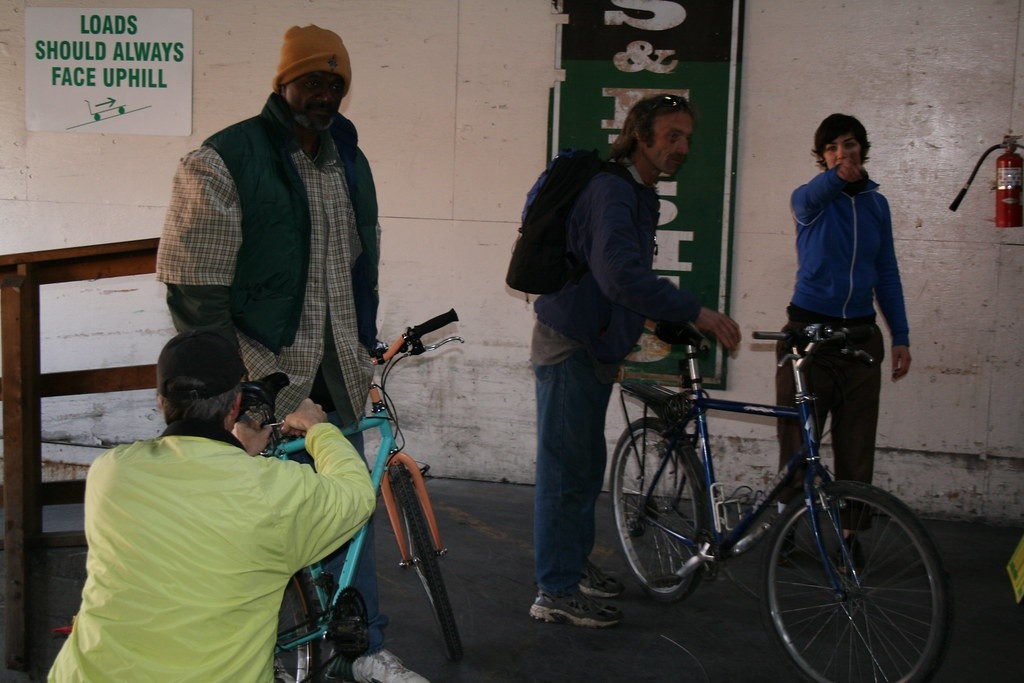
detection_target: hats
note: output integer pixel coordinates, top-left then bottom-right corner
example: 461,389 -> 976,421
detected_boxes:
272,23 -> 351,97
156,330 -> 247,402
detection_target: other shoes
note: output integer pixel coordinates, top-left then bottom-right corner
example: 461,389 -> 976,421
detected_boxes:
840,535 -> 877,588
769,535 -> 797,568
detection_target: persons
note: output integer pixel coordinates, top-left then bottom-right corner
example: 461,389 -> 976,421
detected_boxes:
47,329 -> 376,683
156,26 -> 434,683
775,112 -> 911,573
529,93 -> 742,626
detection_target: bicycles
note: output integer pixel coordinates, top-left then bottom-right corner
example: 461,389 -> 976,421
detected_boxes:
237,307 -> 465,683
609,323 -> 947,683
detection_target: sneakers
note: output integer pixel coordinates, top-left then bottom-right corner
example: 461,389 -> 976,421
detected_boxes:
352,649 -> 430,683
274,657 -> 295,683
531,586 -> 621,629
577,560 -> 620,597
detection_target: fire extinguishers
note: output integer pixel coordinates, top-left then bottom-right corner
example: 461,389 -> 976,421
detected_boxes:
948,131 -> 1024,229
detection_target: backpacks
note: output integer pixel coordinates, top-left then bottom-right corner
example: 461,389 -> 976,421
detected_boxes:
505,148 -> 639,293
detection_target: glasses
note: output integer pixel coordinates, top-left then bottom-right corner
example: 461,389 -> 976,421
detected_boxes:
650,95 -> 691,113
